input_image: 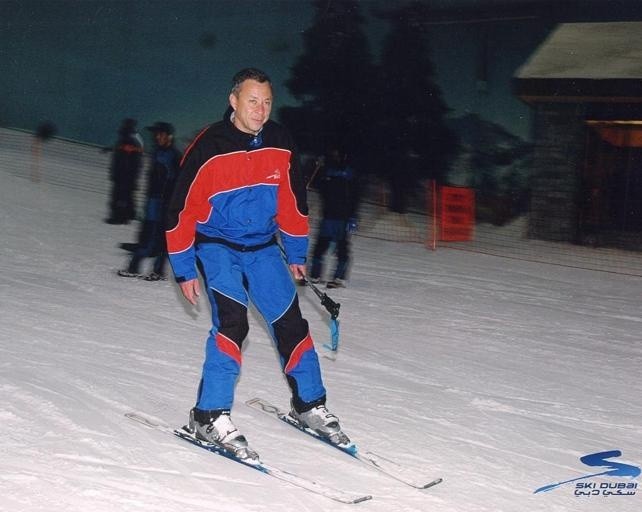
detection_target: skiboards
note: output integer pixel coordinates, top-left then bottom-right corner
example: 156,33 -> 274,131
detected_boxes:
114,270 -> 167,280
125,398 -> 443,503
294,279 -> 350,290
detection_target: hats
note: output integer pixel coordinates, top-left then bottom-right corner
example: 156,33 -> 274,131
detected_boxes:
144,121 -> 176,133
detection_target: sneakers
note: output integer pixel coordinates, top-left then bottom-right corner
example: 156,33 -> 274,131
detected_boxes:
188,408 -> 249,450
302,277 -> 346,288
118,269 -> 164,281
290,396 -> 340,433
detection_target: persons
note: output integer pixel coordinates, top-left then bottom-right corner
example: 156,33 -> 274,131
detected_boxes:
304,147 -> 366,289
103,118 -> 144,224
118,122 -> 183,281
163,67 -> 340,449
